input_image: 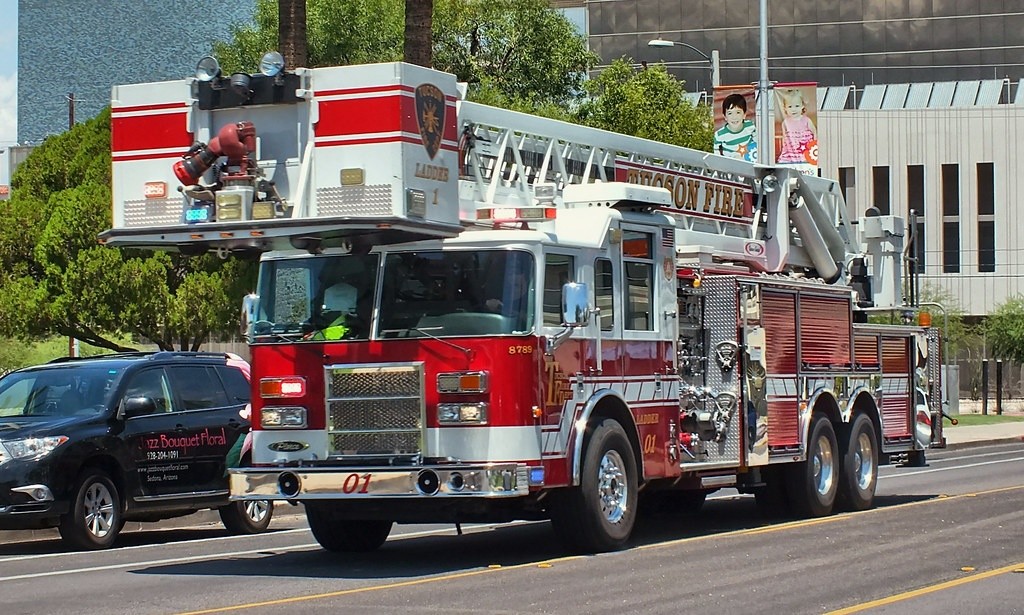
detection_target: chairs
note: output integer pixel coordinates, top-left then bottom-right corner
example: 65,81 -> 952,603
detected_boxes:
60,389 -> 83,414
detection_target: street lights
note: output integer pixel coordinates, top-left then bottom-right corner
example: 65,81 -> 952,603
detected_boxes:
647,37 -> 721,124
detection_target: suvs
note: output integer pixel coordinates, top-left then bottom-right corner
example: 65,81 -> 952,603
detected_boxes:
0,350 -> 277,551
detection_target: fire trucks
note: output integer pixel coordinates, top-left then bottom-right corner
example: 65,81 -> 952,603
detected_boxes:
100,57 -> 961,559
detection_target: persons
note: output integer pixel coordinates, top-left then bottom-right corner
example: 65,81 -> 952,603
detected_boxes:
714,94 -> 757,164
777,91 -> 818,163
483,265 -> 529,330
